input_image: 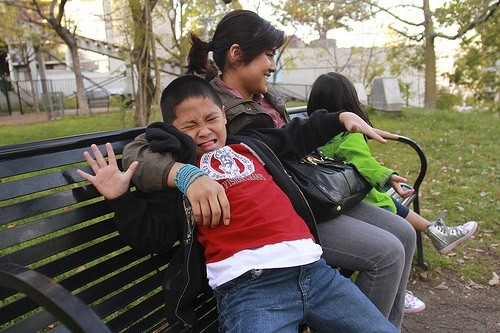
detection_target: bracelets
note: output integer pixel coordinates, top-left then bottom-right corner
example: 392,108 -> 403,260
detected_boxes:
172,163 -> 208,194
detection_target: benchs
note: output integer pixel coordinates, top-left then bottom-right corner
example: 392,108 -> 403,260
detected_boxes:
73,91 -> 112,116
0,105 -> 429,333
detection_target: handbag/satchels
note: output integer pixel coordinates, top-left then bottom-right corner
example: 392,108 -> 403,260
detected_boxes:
281,153 -> 373,223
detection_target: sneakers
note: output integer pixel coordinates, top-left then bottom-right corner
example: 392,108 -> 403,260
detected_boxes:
403,290 -> 425,313
423,218 -> 477,255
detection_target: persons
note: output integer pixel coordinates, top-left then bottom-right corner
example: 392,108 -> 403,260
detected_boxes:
123,10 -> 418,333
306,72 -> 475,313
76,75 -> 397,333
